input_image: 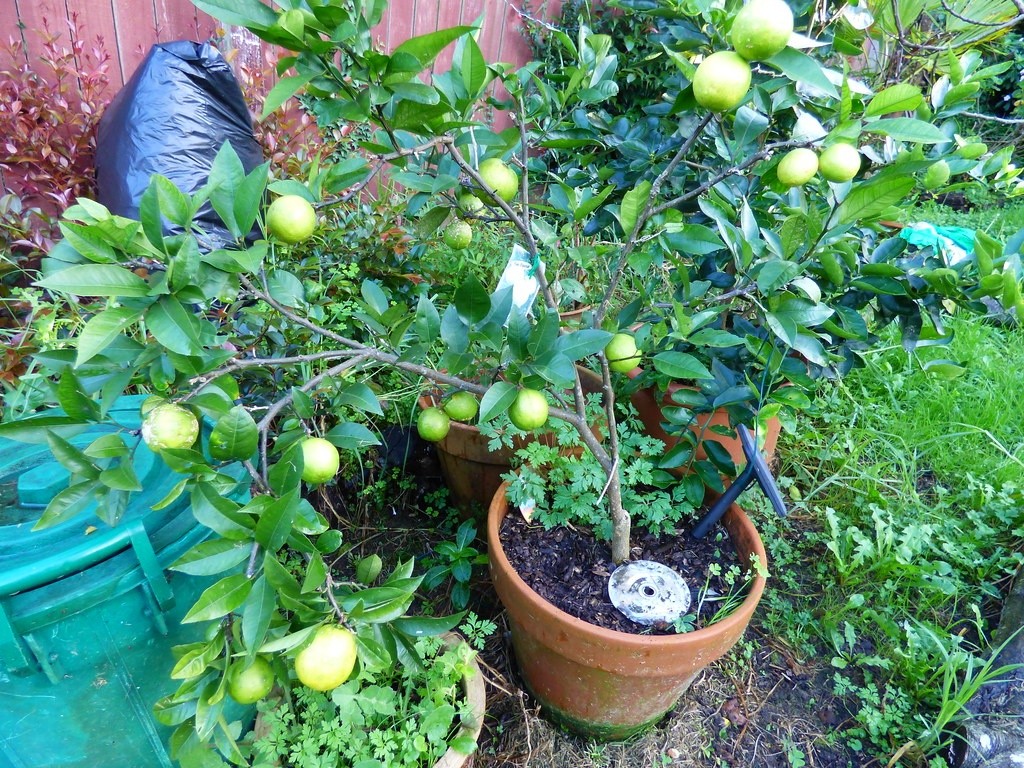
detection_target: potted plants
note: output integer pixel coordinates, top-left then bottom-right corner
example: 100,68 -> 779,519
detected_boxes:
0,0 -> 1023,768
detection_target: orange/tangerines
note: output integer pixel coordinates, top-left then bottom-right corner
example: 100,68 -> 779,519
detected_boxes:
142,0 -> 862,702
